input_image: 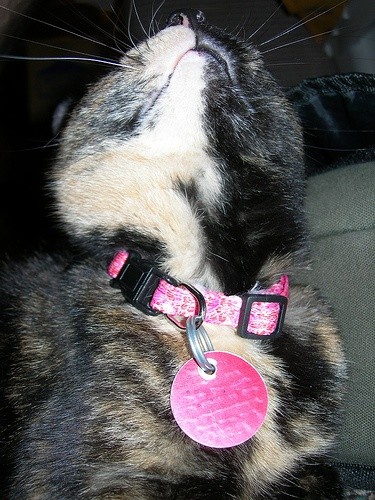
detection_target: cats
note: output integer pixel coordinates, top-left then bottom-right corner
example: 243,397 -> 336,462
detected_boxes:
0,1 -> 352,500
